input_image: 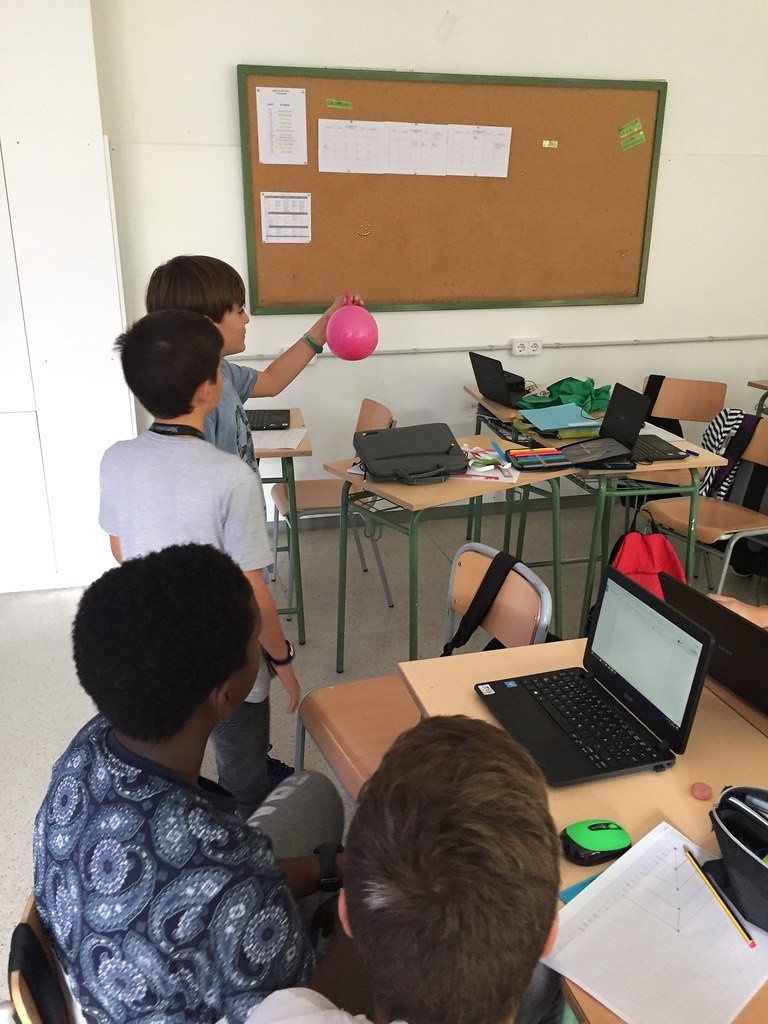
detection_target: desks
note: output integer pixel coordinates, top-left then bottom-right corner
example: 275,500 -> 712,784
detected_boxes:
248,406 -> 314,645
322,432 -> 583,674
397,635 -> 768,1024
462,382 -> 728,641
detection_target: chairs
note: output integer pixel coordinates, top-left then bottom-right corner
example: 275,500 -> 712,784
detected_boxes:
10,886 -> 89,1024
270,397 -> 396,620
642,416 -> 768,597
610,374 -> 729,533
295,541 -> 554,806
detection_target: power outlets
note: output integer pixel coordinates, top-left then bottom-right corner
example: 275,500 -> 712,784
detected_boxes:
509,339 -> 543,355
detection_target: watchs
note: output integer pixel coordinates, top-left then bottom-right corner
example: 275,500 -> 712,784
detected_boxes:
313,842 -> 344,893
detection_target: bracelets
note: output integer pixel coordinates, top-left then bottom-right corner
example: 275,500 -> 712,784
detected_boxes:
302,332 -> 323,354
267,639 -> 295,665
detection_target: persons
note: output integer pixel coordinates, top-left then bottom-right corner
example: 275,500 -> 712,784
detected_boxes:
98,308 -> 301,820
146,255 -> 365,581
30,542 -> 346,1024
246,714 -> 560,1024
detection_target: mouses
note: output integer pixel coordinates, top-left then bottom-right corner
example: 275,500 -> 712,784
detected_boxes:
558,819 -> 631,866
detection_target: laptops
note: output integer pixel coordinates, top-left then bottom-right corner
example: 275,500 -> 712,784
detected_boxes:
598,383 -> 689,462
468,351 -> 538,409
244,410 -> 291,430
659,572 -> 768,719
473,565 -> 715,787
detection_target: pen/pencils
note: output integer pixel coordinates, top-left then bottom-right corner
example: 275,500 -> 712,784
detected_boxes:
509,447 -> 573,468
450,474 -> 499,480
686,450 -> 699,456
682,844 -> 756,948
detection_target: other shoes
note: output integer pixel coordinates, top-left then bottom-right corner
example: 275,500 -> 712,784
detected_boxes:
267,744 -> 295,788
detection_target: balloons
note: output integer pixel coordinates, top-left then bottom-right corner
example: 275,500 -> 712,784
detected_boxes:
326,291 -> 378,362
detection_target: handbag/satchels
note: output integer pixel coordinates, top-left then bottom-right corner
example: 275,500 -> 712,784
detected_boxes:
353,423 -> 468,485
482,629 -> 564,653
702,786 -> 768,933
516,377 -> 611,413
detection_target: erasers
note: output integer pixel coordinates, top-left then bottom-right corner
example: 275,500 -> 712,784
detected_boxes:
692,782 -> 712,799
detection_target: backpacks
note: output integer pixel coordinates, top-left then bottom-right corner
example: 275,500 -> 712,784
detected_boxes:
587,509 -> 687,636
